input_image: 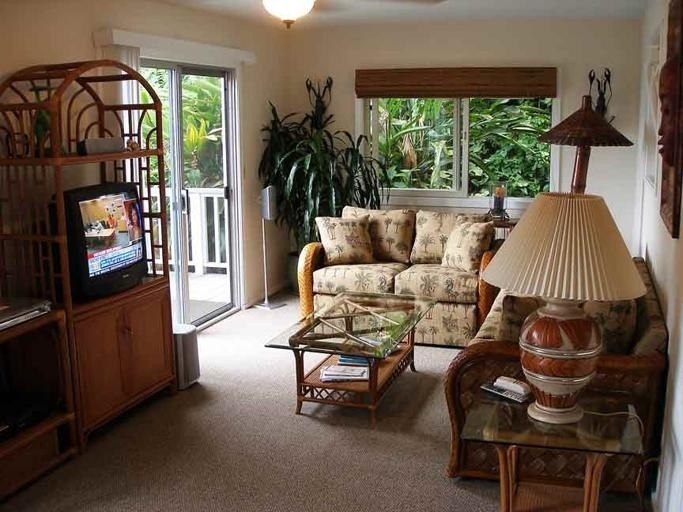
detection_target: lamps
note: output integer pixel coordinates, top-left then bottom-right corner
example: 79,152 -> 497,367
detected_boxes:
262,0 -> 316,28
478,185 -> 649,428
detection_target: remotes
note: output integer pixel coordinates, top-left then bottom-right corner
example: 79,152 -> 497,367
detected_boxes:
494,375 -> 531,395
481,382 -> 528,405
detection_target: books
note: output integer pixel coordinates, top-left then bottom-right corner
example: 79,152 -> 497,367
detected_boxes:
319,335 -> 381,383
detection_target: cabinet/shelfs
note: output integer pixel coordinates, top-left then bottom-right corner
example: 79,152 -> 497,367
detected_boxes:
0,60 -> 177,504
2,307 -> 82,506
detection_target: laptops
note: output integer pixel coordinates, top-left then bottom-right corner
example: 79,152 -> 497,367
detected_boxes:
0,296 -> 51,332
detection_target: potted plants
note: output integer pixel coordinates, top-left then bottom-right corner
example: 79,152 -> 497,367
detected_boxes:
257,76 -> 390,295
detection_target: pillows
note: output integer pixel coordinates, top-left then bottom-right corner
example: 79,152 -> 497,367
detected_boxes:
440,214 -> 497,275
409,208 -> 488,264
341,206 -> 416,264
492,294 -> 540,346
313,212 -> 381,267
581,297 -> 638,354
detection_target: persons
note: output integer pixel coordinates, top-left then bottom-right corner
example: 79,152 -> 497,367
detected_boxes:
93,203 -> 126,229
128,203 -> 143,240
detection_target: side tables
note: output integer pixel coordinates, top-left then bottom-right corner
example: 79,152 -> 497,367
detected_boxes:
459,388 -> 648,511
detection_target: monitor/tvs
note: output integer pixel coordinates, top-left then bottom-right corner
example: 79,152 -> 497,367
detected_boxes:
62,181 -> 148,305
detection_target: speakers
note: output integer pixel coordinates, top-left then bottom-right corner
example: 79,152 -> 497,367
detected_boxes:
261,186 -> 277,220
172,323 -> 201,390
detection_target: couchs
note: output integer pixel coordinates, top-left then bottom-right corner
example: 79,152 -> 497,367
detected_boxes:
298,206 -> 507,349
443,253 -> 667,496
